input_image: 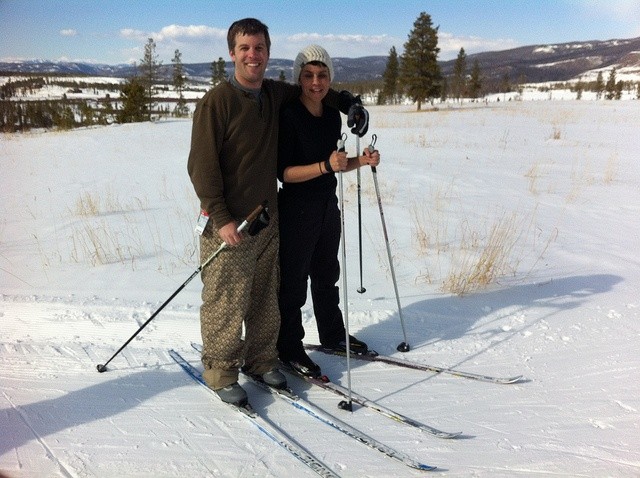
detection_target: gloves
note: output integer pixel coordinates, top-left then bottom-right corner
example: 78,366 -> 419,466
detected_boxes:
340,90 -> 369,137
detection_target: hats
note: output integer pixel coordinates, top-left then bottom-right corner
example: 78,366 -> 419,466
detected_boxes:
293,45 -> 335,86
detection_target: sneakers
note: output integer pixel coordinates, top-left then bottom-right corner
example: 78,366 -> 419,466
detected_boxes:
277,353 -> 322,376
255,370 -> 286,387
321,335 -> 368,354
215,381 -> 248,402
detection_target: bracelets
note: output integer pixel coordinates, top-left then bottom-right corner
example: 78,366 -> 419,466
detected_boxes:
325,159 -> 334,174
316,160 -> 324,174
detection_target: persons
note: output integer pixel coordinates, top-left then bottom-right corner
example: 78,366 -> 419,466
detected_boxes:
187,17 -> 369,407
276,44 -> 380,379
